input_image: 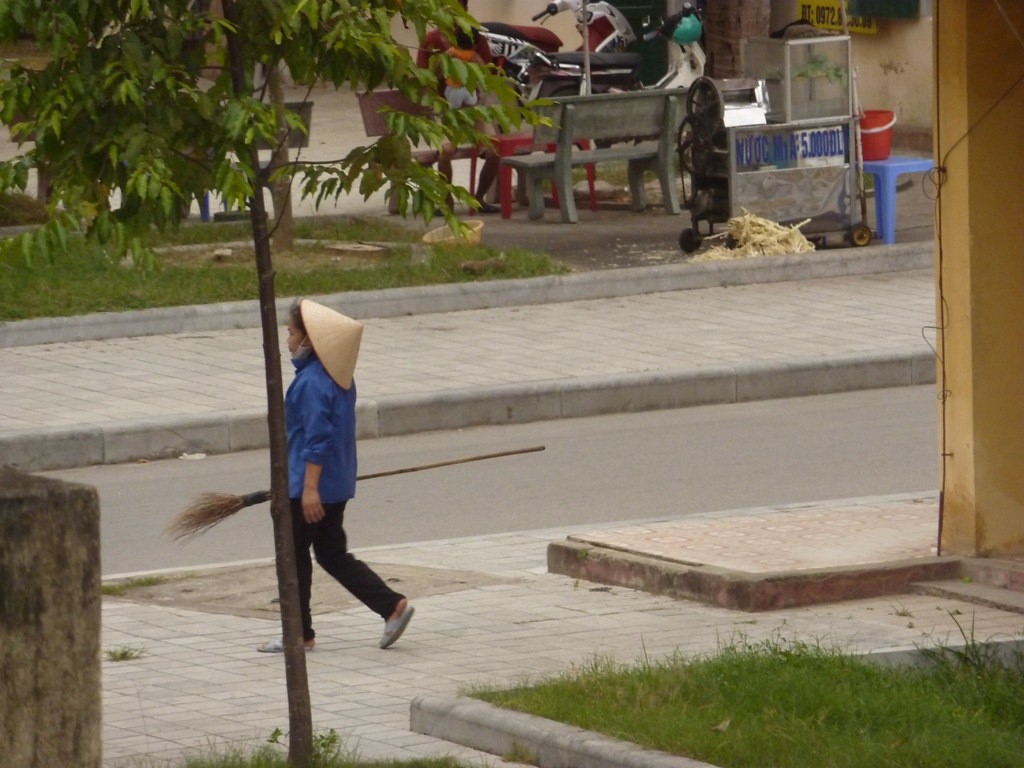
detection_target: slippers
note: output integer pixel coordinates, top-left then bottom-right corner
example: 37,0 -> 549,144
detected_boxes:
379,607 -> 415,648
258,639 -> 313,653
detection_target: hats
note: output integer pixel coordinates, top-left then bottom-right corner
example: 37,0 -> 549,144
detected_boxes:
300,298 -> 363,391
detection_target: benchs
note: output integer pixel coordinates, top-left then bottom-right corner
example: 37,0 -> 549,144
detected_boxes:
355,89 -> 543,214
503,87 -> 697,223
35,99 -> 313,224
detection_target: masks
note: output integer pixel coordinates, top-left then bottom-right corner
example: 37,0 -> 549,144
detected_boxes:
291,334 -> 312,359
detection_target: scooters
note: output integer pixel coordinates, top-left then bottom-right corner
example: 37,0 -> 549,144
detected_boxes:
524,4 -> 709,151
474,0 -> 647,134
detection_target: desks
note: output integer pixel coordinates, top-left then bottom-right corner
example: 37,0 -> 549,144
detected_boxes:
470,132 -> 598,218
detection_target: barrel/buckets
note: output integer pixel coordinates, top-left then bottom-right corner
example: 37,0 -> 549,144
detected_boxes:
854,110 -> 897,161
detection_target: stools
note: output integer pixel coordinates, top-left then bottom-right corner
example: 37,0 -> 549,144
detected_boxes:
854,153 -> 936,245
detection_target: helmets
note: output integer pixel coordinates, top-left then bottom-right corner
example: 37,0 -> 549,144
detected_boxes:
661,6 -> 704,45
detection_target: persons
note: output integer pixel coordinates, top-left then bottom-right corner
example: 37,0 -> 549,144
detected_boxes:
416,0 -> 503,217
255,303 -> 415,652
433,28 -> 486,111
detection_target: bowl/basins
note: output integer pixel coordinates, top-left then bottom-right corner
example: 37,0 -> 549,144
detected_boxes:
715,77 -> 757,101
